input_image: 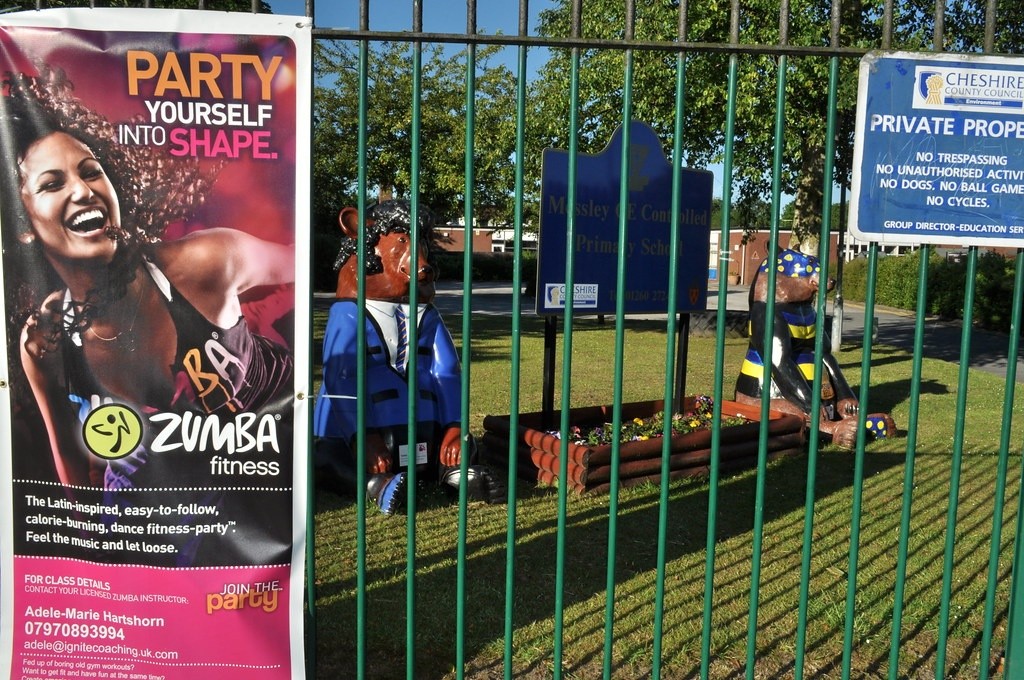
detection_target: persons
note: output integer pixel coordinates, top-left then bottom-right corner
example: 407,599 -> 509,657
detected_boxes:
0,63 -> 294,551
